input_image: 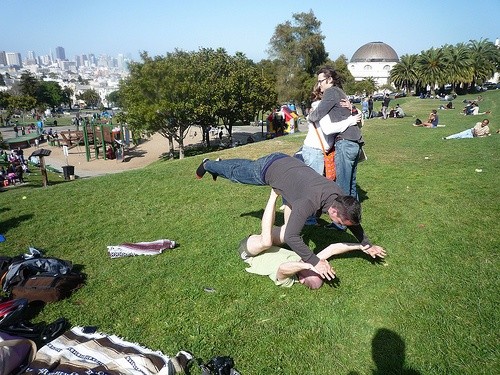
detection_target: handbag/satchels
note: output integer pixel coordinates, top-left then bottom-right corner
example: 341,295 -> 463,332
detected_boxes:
325,151 -> 336,180
12,272 -> 83,301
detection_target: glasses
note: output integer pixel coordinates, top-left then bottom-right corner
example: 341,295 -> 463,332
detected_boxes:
317,76 -> 330,83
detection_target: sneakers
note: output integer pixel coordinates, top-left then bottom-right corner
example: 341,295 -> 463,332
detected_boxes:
324,223 -> 347,232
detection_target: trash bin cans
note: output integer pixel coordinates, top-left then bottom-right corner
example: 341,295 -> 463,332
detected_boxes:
62,166 -> 74,180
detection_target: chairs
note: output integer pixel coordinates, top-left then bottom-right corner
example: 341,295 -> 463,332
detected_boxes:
9,175 -> 20,184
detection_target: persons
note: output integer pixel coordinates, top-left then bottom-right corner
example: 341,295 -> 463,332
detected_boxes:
444,119 -> 491,140
247,136 -> 254,143
413,110 -> 438,128
302,81 -> 363,225
382,93 -> 391,120
219,131 -> 223,139
420,89 -> 456,101
368,96 -> 373,118
463,95 -> 486,116
238,188 -> 371,289
0,147 -> 32,184
309,67 -> 365,232
0,110 -> 59,148
195,152 -> 386,281
106,146 -> 116,159
194,132 -> 197,137
364,102 -> 404,119
71,113 -> 100,127
439,102 -> 452,109
